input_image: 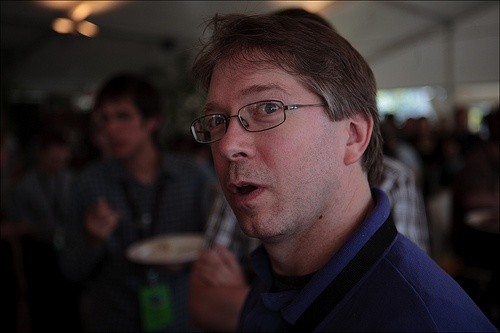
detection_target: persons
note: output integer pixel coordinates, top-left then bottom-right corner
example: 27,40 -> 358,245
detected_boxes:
189,6 -> 432,333
187,12 -> 500,333
0,70 -> 500,333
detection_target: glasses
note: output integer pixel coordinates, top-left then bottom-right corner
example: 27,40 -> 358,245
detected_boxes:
98,111 -> 142,123
190,100 -> 327,144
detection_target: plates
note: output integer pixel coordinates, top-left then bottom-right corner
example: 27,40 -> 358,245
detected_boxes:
123,232 -> 206,265
